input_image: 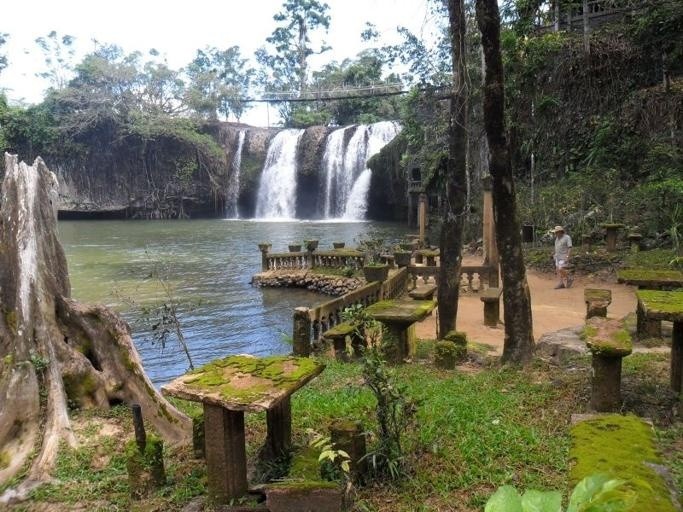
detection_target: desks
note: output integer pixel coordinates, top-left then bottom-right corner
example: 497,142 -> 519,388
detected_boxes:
600,223 -> 624,252
614,270 -> 682,339
158,351 -> 326,507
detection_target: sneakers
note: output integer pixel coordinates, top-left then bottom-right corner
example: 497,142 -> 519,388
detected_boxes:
566,279 -> 573,288
554,283 -> 564,288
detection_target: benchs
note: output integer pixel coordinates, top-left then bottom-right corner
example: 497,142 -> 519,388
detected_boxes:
567,288 -> 683,512
480,287 -> 503,327
415,246 -> 439,267
627,233 -> 642,253
323,283 -> 467,371
582,235 -> 592,253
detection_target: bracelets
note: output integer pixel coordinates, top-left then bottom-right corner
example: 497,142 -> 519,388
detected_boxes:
566,255 -> 569,258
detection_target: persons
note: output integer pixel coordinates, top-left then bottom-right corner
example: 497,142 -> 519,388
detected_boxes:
550,224 -> 574,289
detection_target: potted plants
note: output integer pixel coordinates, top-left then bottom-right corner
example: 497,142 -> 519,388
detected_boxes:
356,225 -> 390,280
394,238 -> 412,267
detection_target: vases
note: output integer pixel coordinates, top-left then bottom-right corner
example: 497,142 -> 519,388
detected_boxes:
289,245 -> 301,252
258,243 -> 272,252
333,243 -> 345,248
306,240 -> 319,248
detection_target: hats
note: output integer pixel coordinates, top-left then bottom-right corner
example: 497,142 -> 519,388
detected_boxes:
550,226 -> 565,233
